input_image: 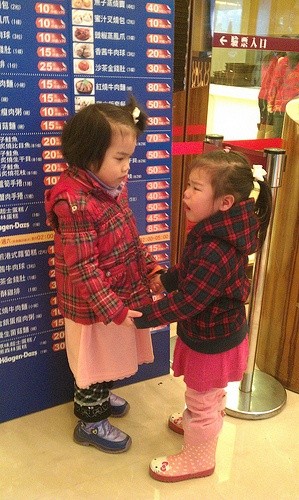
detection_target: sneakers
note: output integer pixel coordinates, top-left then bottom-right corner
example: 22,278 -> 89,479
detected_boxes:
106,390 -> 130,415
72,417 -> 132,453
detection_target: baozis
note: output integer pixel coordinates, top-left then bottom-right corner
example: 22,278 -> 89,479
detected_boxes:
73,11 -> 92,24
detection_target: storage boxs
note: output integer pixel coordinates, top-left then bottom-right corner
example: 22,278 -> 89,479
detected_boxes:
210,62 -> 262,87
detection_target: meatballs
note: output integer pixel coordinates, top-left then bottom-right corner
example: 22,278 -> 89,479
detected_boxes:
75,44 -> 92,57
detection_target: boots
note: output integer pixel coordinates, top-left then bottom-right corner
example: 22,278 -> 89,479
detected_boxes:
147,434 -> 217,483
166,408 -> 227,437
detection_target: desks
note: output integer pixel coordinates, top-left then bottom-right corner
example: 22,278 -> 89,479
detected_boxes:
206,84 -> 266,140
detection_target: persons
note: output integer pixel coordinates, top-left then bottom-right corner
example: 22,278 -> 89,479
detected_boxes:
256,35 -> 290,139
46,96 -> 165,454
265,35 -> 299,138
132,147 -> 275,483
